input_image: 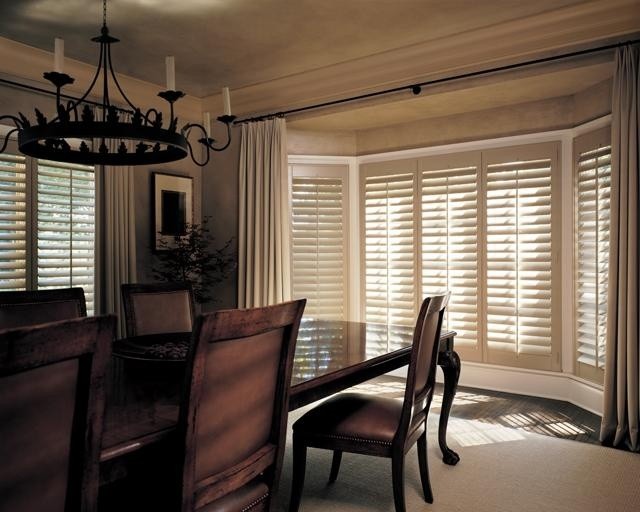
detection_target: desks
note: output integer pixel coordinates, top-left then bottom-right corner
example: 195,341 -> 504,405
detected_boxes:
99,319 -> 461,465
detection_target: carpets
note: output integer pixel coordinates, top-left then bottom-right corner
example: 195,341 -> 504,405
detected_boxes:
280,399 -> 640,512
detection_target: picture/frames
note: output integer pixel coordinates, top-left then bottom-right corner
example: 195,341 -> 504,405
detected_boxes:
151,171 -> 194,252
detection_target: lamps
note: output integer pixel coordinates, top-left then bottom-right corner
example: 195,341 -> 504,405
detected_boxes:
0,0 -> 237,167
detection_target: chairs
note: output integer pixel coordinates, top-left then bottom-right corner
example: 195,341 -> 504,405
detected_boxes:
285,292 -> 451,512
121,279 -> 196,339
140,300 -> 306,511
0,287 -> 87,330
0,314 -> 118,512
